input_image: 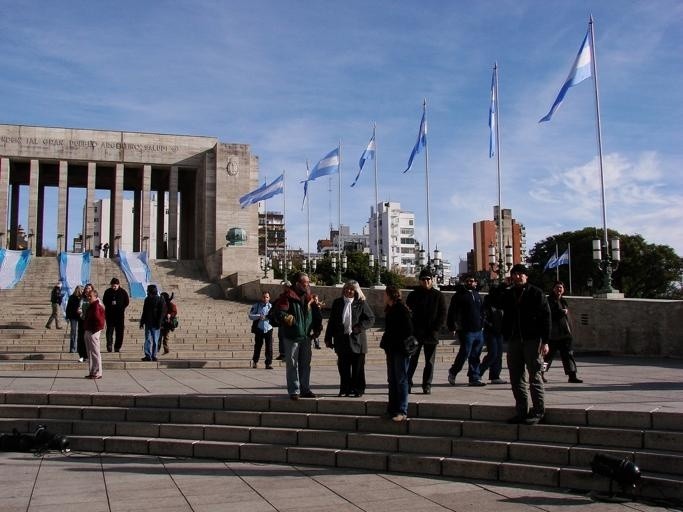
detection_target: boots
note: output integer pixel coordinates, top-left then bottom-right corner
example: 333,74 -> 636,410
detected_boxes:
568,373 -> 582,383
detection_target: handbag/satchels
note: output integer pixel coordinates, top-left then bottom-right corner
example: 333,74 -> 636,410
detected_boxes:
403,335 -> 418,355
251,303 -> 258,333
588,453 -> 641,503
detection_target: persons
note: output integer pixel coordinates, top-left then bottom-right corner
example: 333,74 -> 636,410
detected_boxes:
65,277 -> 177,379
447,273 -> 508,385
541,282 -> 583,384
380,285 -> 415,421
405,268 -> 447,393
249,290 -> 275,370
103,243 -> 109,258
274,271 -> 325,401
492,264 -> 552,427
45,281 -> 65,329
325,279 -> 375,398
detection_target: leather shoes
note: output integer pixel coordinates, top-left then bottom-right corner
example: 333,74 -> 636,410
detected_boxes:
423,388 -> 430,394
290,394 -> 298,400
300,390 -> 317,398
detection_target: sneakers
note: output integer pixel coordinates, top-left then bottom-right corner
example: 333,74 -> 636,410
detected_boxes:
78,357 -> 83,362
524,409 -> 544,424
89,375 -> 102,379
447,370 -> 507,386
391,414 -> 407,421
141,358 -> 150,361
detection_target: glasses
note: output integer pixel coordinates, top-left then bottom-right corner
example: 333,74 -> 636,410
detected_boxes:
419,278 -> 430,281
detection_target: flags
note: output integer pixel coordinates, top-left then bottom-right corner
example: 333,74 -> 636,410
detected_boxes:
300,148 -> 339,184
239,183 -> 266,210
402,111 -> 426,175
488,69 -> 498,158
549,248 -> 569,268
538,32 -> 592,124
544,252 -> 557,270
350,134 -> 375,188
301,167 -> 308,211
251,175 -> 283,204
